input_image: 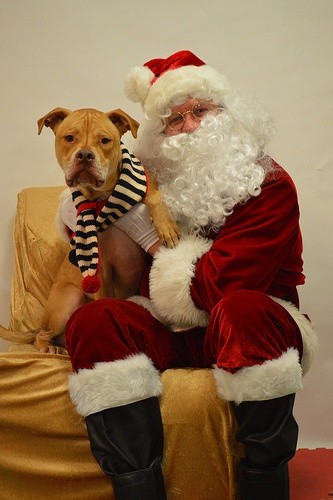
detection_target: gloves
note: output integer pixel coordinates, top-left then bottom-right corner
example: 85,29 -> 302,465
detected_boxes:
60,197 -> 78,232
113,202 -> 160,252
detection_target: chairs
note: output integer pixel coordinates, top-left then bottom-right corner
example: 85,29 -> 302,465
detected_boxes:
1,186 -> 239,500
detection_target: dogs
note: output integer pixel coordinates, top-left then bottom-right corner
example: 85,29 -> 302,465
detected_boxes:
0,106 -> 181,355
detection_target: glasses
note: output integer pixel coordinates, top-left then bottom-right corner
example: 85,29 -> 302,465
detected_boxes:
165,100 -> 212,131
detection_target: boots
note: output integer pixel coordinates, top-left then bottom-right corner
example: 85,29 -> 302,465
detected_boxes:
65,351 -> 167,499
212,347 -> 302,500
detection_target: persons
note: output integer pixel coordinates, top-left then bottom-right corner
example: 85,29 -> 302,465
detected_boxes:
56,50 -> 319,500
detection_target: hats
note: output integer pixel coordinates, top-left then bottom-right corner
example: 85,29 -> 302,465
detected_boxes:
123,53 -> 220,120
